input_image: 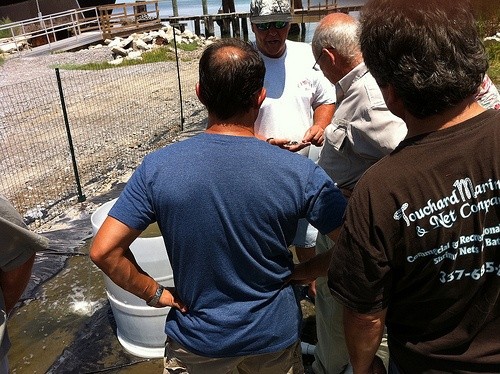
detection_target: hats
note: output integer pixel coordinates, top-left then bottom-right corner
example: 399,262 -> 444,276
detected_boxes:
250,0 -> 294,22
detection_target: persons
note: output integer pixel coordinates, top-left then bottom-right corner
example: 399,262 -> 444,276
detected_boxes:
87,39 -> 347,374
0,194 -> 50,374
327,0 -> 500,374
312,12 -> 408,374
475,72 -> 500,110
247,0 -> 337,304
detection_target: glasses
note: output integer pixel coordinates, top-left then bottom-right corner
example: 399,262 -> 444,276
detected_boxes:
312,49 -> 332,71
255,22 -> 288,30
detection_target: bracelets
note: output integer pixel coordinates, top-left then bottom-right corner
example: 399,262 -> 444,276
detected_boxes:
146,285 -> 165,307
266,138 -> 274,144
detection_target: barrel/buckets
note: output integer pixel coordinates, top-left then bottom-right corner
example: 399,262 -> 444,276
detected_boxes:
90,198 -> 176,358
